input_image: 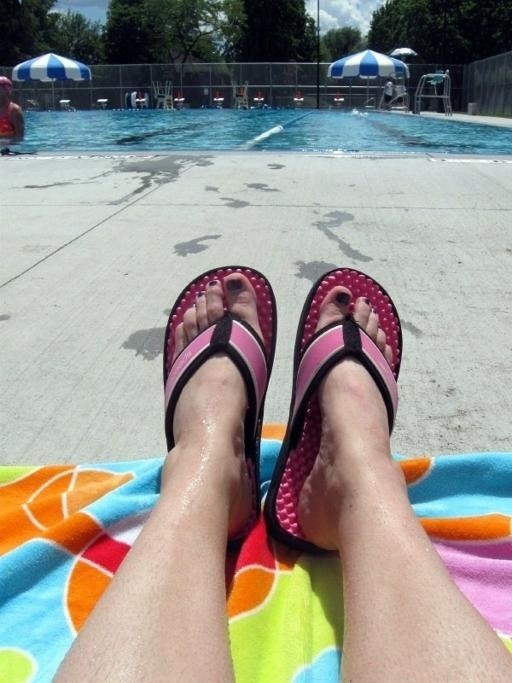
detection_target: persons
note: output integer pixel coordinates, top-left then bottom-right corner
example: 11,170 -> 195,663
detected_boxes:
1,75 -> 27,145
50,263 -> 510,682
382,78 -> 394,108
395,79 -> 407,105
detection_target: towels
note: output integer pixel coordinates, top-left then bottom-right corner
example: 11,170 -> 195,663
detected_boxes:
0,419 -> 512,682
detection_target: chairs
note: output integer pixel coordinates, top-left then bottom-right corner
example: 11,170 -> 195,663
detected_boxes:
23,79 -> 274,109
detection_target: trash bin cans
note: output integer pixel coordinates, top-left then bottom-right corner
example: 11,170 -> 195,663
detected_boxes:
468,103 -> 479,115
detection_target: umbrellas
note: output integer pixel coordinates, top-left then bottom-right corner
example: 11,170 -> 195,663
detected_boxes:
325,48 -> 409,107
11,52 -> 92,111
388,47 -> 418,57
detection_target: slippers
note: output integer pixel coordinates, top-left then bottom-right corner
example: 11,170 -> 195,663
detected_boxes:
163,263 -> 277,539
264,267 -> 401,555
